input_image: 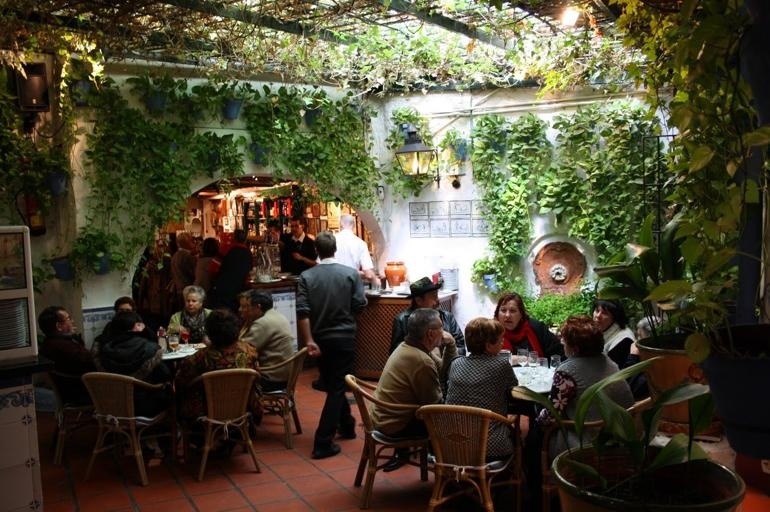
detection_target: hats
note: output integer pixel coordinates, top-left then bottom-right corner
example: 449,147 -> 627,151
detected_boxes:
404,277 -> 443,301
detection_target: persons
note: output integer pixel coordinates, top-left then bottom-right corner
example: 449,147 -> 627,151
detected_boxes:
384,278 -> 466,471
136,228 -> 254,331
297,232 -> 369,459
167,286 -> 213,344
37,296 -> 179,466
536,315 -> 634,477
592,297 -> 677,441
316,214 -> 382,292
494,292 -> 566,365
175,310 -> 264,428
370,307 -> 460,465
278,216 -> 318,277
233,288 -> 293,392
444,317 -> 518,495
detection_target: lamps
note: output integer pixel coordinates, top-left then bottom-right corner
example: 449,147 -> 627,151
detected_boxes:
395,123 -> 433,176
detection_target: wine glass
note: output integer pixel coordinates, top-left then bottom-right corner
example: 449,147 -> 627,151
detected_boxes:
517,348 -> 563,387
180,327 -> 190,349
167,336 -> 179,357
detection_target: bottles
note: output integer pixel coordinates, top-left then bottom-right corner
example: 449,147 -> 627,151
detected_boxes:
244,197 -> 293,239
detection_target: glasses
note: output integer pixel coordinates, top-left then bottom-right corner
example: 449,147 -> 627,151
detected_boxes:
60,315 -> 71,324
430,324 -> 444,329
289,224 -> 304,228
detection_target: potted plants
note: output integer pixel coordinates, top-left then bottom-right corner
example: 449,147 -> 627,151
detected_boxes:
510,354 -> 746,512
593,210 -> 739,423
471,249 -> 510,295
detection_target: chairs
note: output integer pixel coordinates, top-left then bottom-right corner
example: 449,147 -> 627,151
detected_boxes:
81,372 -> 172,488
243,346 -> 308,453
38,351 -> 125,465
183,368 -> 263,482
538,395 -> 667,511
414,404 -> 523,512
344,374 -> 437,509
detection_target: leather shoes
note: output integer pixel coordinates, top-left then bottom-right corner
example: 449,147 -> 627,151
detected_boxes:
336,428 -> 356,439
383,452 -> 409,471
312,444 -> 341,459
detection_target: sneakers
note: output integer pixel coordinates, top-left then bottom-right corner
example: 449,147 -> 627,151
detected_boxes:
312,380 -> 328,392
